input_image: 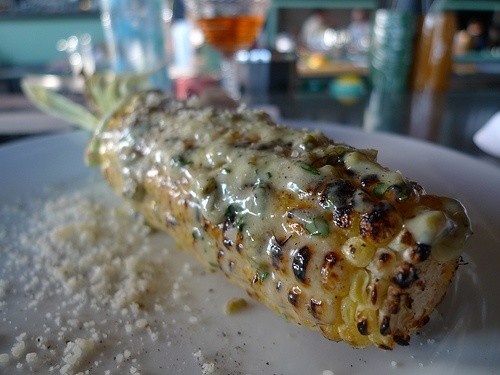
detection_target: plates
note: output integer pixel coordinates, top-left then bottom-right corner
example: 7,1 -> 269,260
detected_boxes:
1,112 -> 500,374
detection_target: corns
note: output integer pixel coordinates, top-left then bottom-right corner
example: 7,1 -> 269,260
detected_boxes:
87,90 -> 475,353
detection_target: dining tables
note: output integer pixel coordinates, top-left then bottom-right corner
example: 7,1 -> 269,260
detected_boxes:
1,91 -> 500,167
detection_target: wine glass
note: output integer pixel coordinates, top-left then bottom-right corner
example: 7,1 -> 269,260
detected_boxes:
186,1 -> 273,110
